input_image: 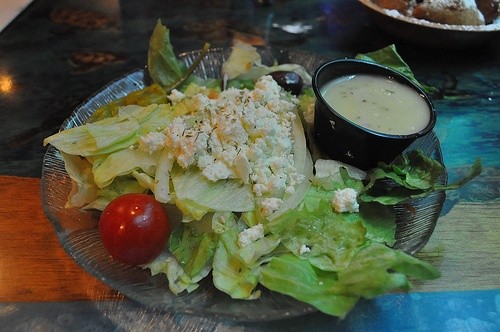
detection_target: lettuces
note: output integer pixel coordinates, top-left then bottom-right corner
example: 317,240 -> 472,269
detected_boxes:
40,19 -> 484,318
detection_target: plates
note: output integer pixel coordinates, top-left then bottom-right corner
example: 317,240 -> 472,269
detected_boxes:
40,45 -> 449,323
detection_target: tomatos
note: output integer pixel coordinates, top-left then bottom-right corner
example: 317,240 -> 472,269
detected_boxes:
99,193 -> 170,265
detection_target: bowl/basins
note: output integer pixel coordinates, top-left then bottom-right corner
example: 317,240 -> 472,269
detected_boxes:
312,60 -> 437,171
357,0 -> 500,50
